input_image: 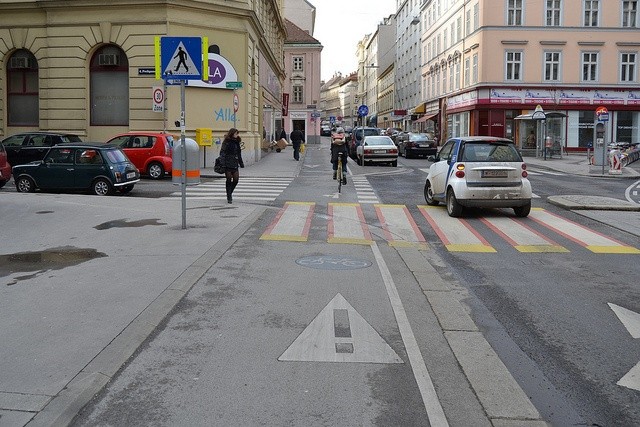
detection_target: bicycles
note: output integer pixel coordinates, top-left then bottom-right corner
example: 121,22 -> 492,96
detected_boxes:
297,256 -> 370,268
607,141 -> 640,168
334,154 -> 344,194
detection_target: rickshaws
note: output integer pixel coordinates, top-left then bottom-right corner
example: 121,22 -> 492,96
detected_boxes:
270,140 -> 286,154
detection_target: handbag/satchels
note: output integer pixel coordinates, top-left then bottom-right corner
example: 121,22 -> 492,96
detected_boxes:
213,157 -> 228,173
299,143 -> 305,153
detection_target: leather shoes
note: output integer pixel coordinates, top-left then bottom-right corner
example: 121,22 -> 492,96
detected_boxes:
228,196 -> 232,204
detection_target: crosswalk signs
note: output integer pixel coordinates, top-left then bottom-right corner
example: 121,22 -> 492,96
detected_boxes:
154,33 -> 210,81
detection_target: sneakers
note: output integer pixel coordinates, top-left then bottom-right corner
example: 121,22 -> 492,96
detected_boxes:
343,174 -> 347,185
333,174 -> 337,180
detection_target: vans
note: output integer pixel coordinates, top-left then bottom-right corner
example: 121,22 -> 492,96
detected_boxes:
350,126 -> 381,160
1,133 -> 83,169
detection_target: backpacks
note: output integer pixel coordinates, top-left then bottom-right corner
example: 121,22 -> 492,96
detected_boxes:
331,132 -> 346,146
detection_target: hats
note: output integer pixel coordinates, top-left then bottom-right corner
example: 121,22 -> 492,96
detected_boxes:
337,127 -> 344,133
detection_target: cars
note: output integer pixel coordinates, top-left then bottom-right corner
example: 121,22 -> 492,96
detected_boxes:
0,141 -> 13,191
381,129 -> 387,136
397,131 -> 437,159
103,133 -> 177,180
345,127 -> 354,142
11,142 -> 142,196
321,125 -> 331,136
387,128 -> 404,146
424,135 -> 532,218
357,136 -> 398,167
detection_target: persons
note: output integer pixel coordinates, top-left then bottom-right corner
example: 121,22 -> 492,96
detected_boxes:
279,128 -> 287,142
329,125 -> 348,185
262,126 -> 266,139
270,127 -> 278,151
213,128 -> 244,203
290,125 -> 305,159
526,130 -> 535,147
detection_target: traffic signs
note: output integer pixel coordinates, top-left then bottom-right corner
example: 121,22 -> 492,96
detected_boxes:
233,93 -> 239,113
311,113 -> 321,118
597,113 -> 609,120
153,79 -> 164,112
226,82 -> 243,89
